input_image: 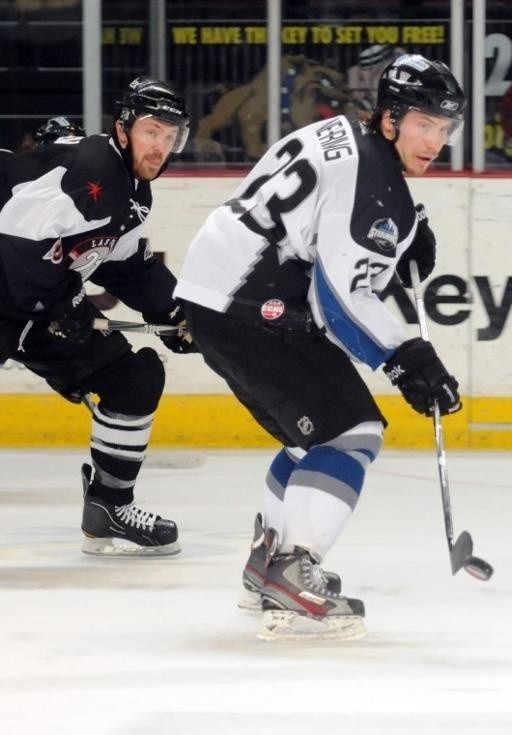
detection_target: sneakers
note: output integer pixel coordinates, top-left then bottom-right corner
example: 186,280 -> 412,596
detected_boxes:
243,513 -> 343,594
78,461 -> 179,547
257,526 -> 368,618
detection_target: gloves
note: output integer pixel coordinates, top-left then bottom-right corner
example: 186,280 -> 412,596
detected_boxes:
150,302 -> 202,356
396,204 -> 438,290
380,336 -> 464,416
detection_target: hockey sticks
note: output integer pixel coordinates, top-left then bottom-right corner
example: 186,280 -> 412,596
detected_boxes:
409,257 -> 473,575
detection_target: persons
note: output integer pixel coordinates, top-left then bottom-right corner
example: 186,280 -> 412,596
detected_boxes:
170,51 -> 464,619
32,115 -> 86,150
0,76 -> 204,547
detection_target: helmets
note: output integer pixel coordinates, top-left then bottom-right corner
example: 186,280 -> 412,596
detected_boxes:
377,54 -> 468,128
116,76 -> 191,134
